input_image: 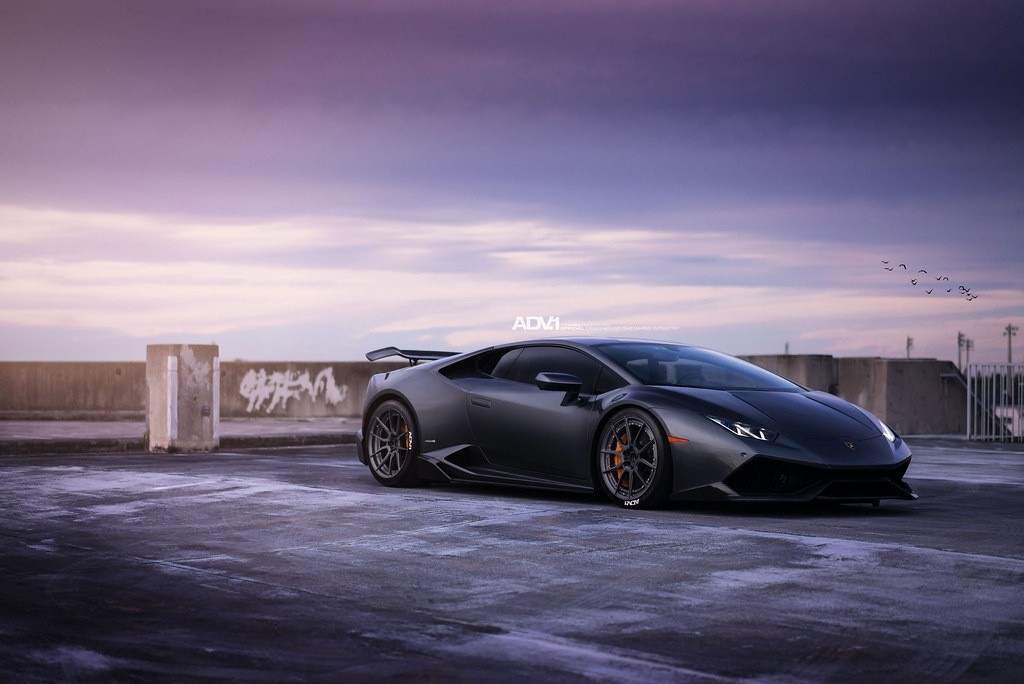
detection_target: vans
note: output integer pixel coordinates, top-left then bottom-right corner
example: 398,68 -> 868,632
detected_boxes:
996,408 -> 1024,440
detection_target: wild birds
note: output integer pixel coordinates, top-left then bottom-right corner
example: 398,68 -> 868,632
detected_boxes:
882,260 -> 978,301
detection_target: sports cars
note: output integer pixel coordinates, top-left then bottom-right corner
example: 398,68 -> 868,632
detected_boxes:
356,336 -> 920,510
